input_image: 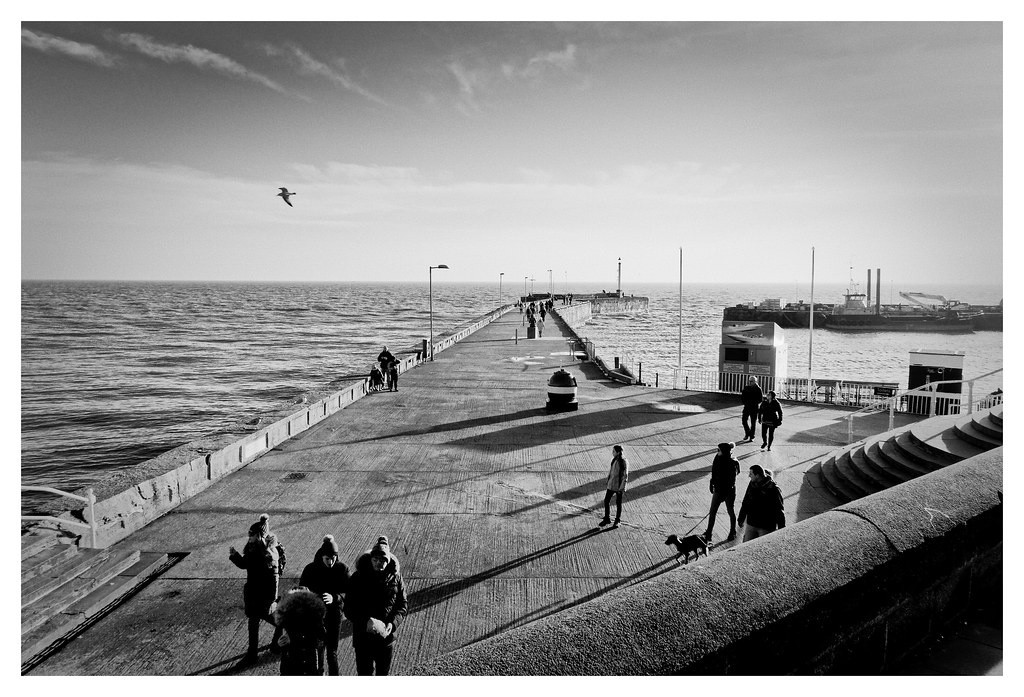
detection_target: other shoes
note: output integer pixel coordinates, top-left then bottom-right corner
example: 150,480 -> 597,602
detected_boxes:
743,431 -> 750,440
702,532 -> 712,541
727,529 -> 736,541
374,385 -> 399,391
236,654 -> 258,669
767,446 -> 771,451
750,438 -> 754,442
761,442 -> 767,449
599,518 -> 611,527
613,518 -> 621,527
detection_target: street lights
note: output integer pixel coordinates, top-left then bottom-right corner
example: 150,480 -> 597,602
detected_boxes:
499,272 -> 504,318
525,276 -> 528,308
429,265 -> 450,361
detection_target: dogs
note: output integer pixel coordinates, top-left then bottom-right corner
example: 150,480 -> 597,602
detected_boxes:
665,534 -> 714,565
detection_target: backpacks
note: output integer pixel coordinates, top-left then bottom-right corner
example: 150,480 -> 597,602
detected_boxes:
262,536 -> 286,577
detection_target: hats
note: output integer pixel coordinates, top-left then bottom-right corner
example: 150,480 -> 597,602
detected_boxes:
371,535 -> 391,564
320,535 -> 339,556
248,514 -> 268,538
718,442 -> 734,456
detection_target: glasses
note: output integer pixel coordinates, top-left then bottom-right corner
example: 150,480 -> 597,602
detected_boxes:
749,380 -> 755,382
717,449 -> 721,452
248,531 -> 258,537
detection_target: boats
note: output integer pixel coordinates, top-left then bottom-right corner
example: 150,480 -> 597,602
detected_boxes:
722,323 -> 765,333
822,265 -> 985,334
725,333 -> 771,346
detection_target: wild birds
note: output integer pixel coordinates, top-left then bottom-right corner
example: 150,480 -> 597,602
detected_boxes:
277,185 -> 296,208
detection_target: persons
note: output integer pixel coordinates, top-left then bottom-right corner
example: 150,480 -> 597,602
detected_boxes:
537,318 -> 544,337
529,314 -> 536,327
299,535 -> 352,676
227,515 -> 281,665
702,442 -> 740,541
737,465 -> 787,544
599,446 -> 627,527
269,585 -> 326,676
342,536 -> 408,676
520,299 -> 553,322
758,391 -> 783,451
739,376 -> 762,442
371,345 -> 400,392
